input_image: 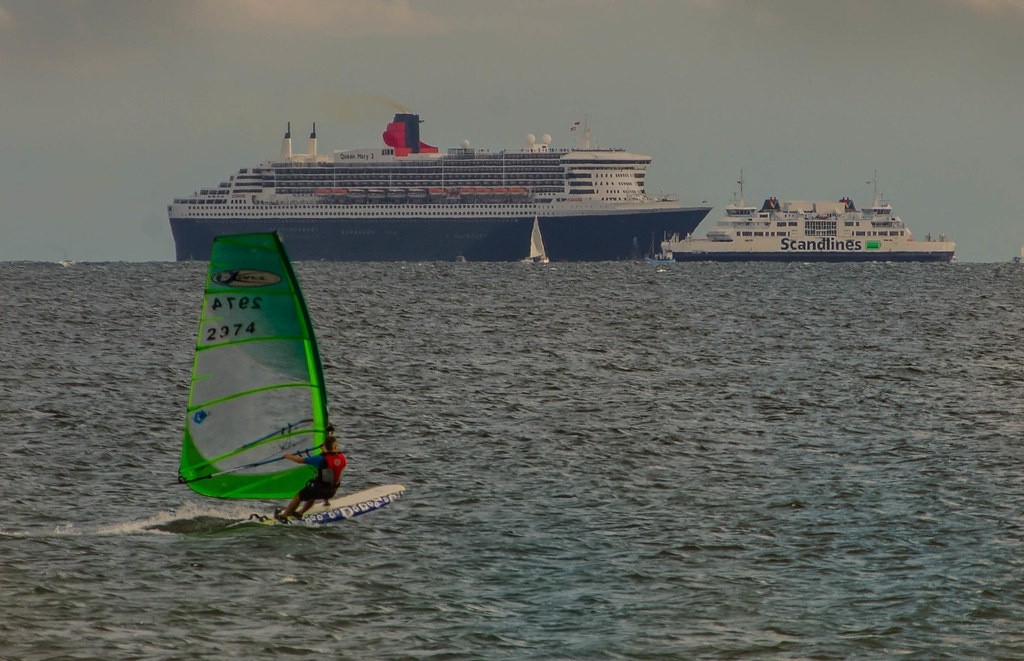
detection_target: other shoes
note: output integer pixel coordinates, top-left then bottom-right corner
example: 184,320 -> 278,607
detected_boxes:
291,511 -> 302,520
274,513 -> 288,524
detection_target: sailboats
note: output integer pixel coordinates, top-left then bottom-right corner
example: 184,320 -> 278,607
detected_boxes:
526,213 -> 551,264
653,231 -> 676,262
177,231 -> 407,529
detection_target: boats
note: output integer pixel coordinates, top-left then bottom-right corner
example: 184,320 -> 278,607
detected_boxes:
658,166 -> 956,262
1011,257 -> 1023,264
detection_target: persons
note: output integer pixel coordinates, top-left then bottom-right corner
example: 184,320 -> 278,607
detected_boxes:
274,436 -> 346,524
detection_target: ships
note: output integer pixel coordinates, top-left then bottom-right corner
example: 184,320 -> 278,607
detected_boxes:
167,112 -> 712,261
